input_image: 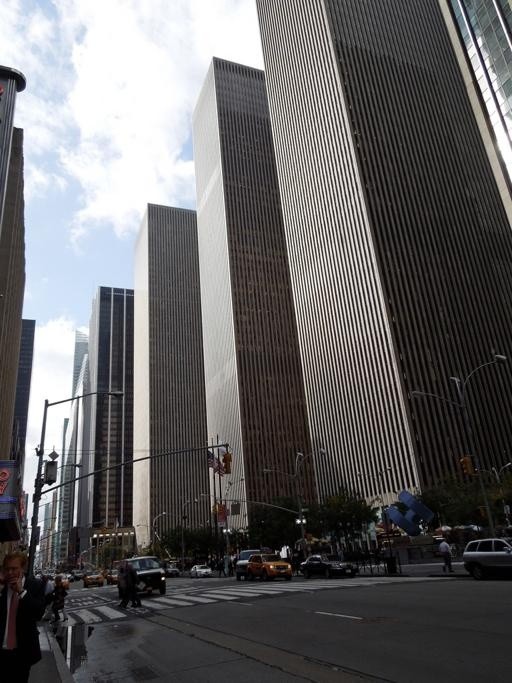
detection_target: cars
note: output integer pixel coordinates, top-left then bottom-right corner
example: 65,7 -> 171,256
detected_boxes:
190,565 -> 212,578
71,569 -> 119,588
298,553 -> 358,579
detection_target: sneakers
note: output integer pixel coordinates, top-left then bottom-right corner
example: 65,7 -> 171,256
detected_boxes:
443,567 -> 445,572
450,571 -> 454,573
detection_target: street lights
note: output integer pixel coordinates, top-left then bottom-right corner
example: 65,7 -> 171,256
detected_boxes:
262,448 -> 324,558
27,390 -> 123,581
475,462 -> 511,530
358,468 -> 393,558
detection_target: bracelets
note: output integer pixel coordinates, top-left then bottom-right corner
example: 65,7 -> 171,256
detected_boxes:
18,589 -> 25,595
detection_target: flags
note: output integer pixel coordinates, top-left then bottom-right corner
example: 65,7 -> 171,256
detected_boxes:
214,457 -> 219,472
208,450 -> 215,467
217,460 -> 228,478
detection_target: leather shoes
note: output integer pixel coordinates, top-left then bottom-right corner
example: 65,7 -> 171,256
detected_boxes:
63,618 -> 68,622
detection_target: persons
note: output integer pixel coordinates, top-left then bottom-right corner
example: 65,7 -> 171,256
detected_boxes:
438,538 -> 455,573
209,554 -> 239,577
1,551 -> 48,682
116,560 -> 142,609
38,572 -> 71,625
286,546 -> 345,578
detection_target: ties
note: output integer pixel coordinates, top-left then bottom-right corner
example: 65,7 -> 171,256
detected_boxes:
7,593 -> 19,651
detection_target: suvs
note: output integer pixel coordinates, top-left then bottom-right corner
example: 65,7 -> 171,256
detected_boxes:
247,554 -> 292,581
118,555 -> 167,598
462,538 -> 512,580
235,549 -> 262,581
